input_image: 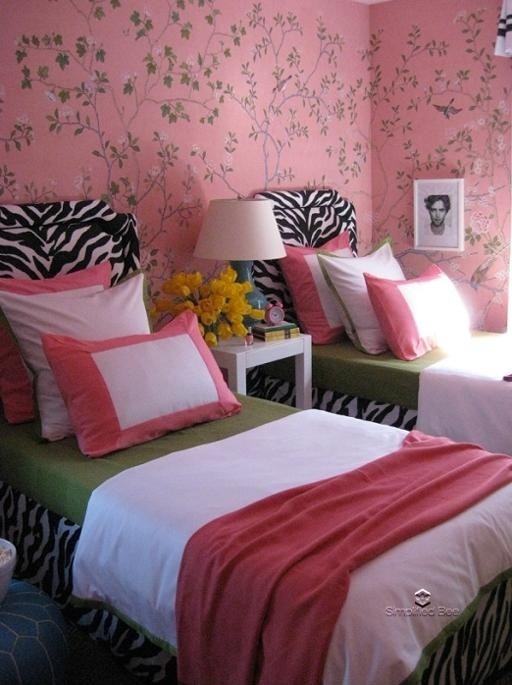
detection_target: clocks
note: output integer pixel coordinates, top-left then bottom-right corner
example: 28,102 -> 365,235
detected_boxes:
263,302 -> 285,327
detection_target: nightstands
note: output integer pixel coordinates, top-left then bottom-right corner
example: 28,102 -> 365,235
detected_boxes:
159,329 -> 314,413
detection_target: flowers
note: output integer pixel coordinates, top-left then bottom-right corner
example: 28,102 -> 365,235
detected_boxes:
147,266 -> 266,348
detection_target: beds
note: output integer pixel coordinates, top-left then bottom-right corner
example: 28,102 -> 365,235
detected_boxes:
0,198 -> 512,685
243,187 -> 512,456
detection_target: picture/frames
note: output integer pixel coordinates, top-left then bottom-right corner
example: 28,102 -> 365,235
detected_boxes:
413,178 -> 464,253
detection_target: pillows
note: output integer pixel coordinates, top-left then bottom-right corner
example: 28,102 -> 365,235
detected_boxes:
314,236 -> 407,357
1,271 -> 152,447
277,229 -> 357,346
363,263 -> 474,360
2,259 -> 116,429
41,308 -> 242,456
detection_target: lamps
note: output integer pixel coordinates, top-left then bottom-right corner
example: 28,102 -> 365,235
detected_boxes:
191,197 -> 288,333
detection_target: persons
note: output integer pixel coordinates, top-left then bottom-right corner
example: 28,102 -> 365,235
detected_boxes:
420,192 -> 458,247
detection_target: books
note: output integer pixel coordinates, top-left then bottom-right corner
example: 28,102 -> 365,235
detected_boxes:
252,324 -> 301,342
